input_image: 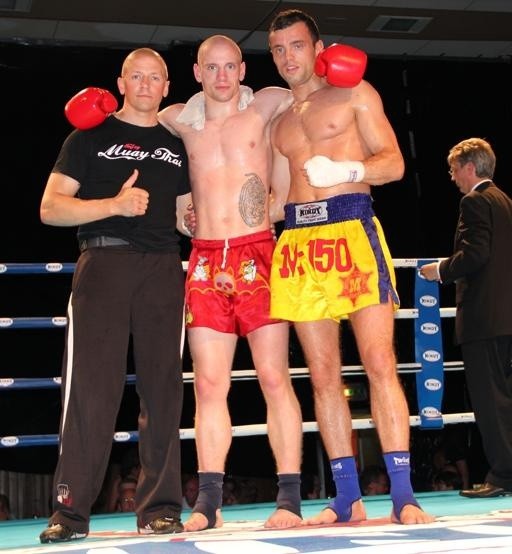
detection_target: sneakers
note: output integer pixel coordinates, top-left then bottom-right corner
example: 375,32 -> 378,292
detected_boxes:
40,522 -> 86,542
137,518 -> 184,534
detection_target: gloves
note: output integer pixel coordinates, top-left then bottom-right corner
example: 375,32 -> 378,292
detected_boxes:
65,88 -> 119,130
315,43 -> 368,88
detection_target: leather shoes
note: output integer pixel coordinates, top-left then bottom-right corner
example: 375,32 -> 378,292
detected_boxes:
460,481 -> 512,498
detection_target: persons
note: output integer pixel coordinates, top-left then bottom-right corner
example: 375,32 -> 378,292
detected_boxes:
357,463 -> 390,495
299,457 -> 321,500
0,494 -> 44,520
65,36 -> 368,531
39,48 -> 196,543
418,137 -> 512,499
409,444 -> 470,491
184,9 -> 444,525
90,455 -> 259,514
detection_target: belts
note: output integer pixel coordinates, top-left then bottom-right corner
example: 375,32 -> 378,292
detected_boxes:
80,238 -> 131,250
